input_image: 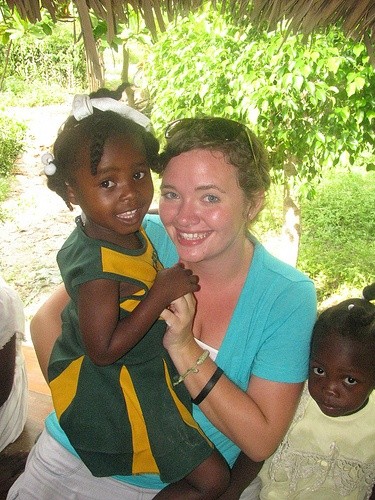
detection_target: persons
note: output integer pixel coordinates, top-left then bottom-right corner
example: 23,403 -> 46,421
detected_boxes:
45,84 -> 233,500
220,281 -> 375,500
31,113 -> 319,500
0,277 -> 26,451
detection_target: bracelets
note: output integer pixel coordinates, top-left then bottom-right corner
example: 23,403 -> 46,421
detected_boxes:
171,349 -> 210,387
191,367 -> 224,406
